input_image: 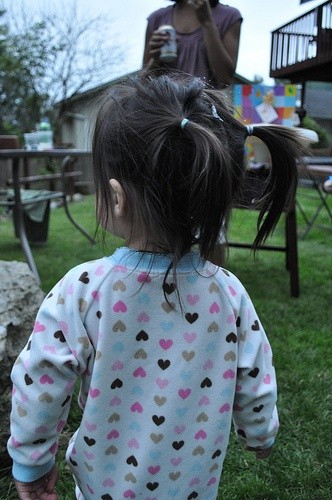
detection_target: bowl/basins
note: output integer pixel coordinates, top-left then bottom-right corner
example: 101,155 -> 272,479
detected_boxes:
247,123 -> 319,163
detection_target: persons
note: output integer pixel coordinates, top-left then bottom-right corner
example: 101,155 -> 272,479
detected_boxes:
6,69 -> 282,499
142,0 -> 243,265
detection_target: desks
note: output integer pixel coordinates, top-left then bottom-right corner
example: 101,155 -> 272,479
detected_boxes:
0,148 -> 98,286
290,154 -> 332,239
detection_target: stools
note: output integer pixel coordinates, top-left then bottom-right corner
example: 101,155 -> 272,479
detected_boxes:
204,160 -> 301,295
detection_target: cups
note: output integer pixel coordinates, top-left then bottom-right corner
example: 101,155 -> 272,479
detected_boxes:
0,134 -> 20,148
24,133 -> 40,150
39,128 -> 53,148
